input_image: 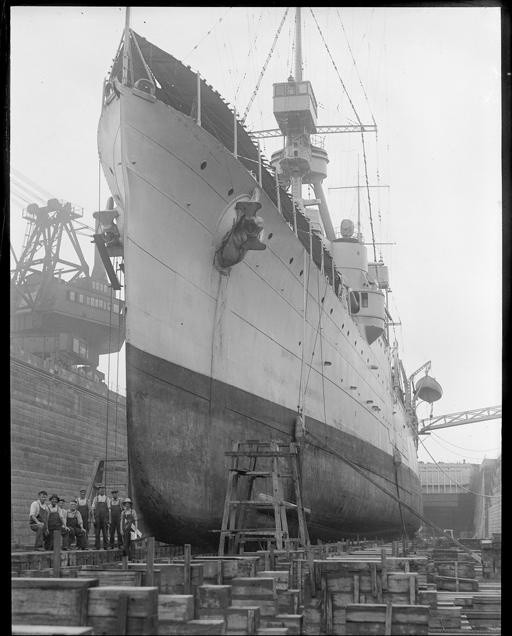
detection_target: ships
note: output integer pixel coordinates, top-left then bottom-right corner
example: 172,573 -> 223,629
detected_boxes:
97,7 -> 424,550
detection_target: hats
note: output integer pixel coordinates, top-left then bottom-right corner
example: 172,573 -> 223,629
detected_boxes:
122,498 -> 133,506
99,487 -> 119,493
80,490 -> 85,494
49,494 -> 65,502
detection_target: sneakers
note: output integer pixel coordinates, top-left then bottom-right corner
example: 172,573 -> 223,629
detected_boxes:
63,546 -> 122,550
34,548 -> 45,552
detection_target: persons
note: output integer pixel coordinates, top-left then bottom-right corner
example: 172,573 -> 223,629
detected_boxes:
29,491 -> 50,552
91,486 -> 112,550
119,497 -> 138,555
67,500 -> 89,550
58,498 -> 67,526
109,490 -> 125,549
48,494 -> 70,551
75,489 -> 92,550
